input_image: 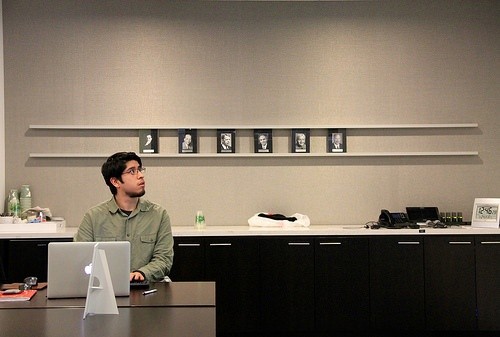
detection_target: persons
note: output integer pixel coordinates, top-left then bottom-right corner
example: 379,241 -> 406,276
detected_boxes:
143,134 -> 154,152
73,152 -> 174,282
221,134 -> 231,150
182,133 -> 193,150
332,133 -> 343,149
295,133 -> 306,149
258,134 -> 269,150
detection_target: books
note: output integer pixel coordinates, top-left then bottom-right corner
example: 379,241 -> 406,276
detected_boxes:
0,290 -> 37,301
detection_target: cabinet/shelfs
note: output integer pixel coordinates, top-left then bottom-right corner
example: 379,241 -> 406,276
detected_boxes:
0,225 -> 500,337
29,123 -> 478,157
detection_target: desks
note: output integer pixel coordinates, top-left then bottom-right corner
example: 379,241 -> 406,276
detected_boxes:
0,282 -> 215,337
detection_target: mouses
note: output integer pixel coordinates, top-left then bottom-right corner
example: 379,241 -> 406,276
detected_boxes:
24,277 -> 38,287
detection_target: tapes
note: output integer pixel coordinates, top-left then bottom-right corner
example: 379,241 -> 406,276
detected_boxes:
419,230 -> 425,233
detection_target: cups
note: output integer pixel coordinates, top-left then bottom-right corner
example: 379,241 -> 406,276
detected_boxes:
27,210 -> 38,222
37,211 -> 46,222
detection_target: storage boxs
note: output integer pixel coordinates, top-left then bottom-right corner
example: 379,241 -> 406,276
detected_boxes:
0,221 -> 66,233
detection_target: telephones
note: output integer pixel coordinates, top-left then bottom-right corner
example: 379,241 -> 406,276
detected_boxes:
379,208 -> 412,228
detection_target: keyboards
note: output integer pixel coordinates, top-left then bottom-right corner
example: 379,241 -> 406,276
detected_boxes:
130,280 -> 150,289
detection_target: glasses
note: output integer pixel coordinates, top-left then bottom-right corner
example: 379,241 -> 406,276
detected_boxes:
121,167 -> 146,175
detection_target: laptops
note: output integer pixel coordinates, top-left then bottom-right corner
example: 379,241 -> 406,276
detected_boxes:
47,241 -> 130,299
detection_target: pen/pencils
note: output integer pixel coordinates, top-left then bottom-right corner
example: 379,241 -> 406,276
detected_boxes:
143,289 -> 158,295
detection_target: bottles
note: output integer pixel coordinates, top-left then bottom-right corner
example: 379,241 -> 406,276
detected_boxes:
196,210 -> 205,229
19,185 -> 32,219
5,189 -> 19,216
440,212 -> 463,223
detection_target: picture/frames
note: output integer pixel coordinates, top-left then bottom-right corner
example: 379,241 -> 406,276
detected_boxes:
179,129 -> 198,153
292,128 -> 311,153
254,129 -> 272,153
139,129 -> 158,153
217,129 -> 236,153
329,128 -> 346,153
